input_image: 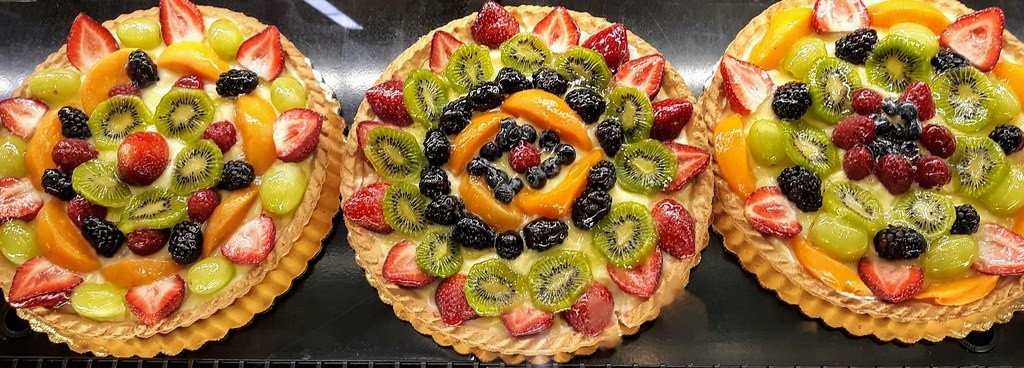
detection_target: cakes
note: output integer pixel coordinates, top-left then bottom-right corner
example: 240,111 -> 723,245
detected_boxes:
0,0 -> 346,358
692,0 -> 1024,343
339,0 -> 715,364
